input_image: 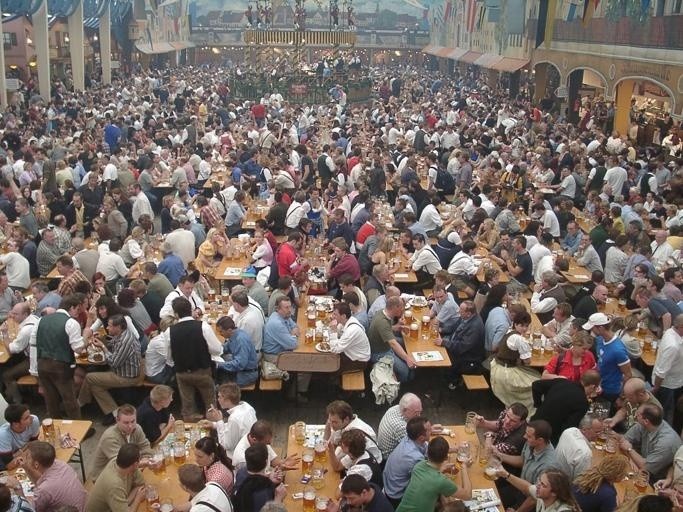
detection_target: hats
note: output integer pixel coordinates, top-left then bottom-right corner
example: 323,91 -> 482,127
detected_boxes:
239,272 -> 256,278
582,312 -> 610,330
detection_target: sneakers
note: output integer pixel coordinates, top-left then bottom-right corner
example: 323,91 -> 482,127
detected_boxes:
448,379 -> 464,389
102,413 -> 115,426
82,428 -> 95,441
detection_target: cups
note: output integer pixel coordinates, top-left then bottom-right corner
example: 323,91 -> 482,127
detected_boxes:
443,411 -> 501,480
589,399 -> 649,494
0,230 -> 660,365
39,417 -> 59,441
288,421 -> 329,512
144,419 -> 201,512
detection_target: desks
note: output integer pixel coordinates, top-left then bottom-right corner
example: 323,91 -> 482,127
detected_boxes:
0,165 -> 682,507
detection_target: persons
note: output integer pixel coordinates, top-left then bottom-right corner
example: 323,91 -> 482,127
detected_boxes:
330,5 -> 339,24
264,5 -> 271,28
292,4 -> 303,29
245,5 -> 253,24
346,6 -> 353,26
255,1 -> 265,24
